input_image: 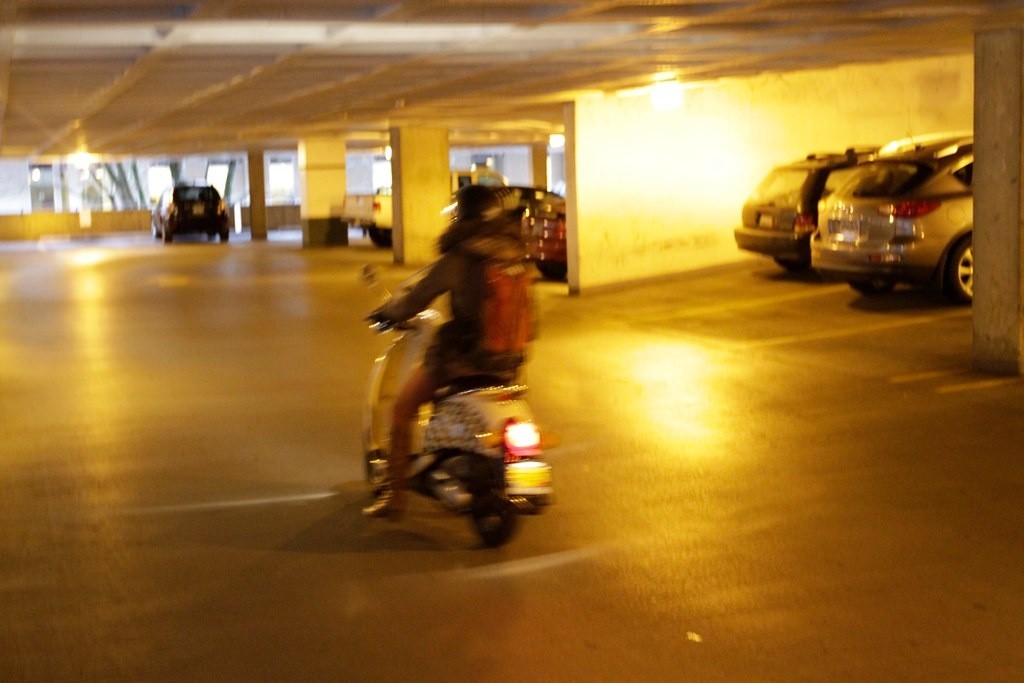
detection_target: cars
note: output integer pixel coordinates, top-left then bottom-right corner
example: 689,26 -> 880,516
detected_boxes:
496,185 -> 565,245
520,199 -> 566,278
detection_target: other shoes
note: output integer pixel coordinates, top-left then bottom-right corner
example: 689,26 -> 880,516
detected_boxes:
361,486 -> 401,517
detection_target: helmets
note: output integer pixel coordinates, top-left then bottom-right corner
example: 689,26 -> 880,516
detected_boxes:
455,185 -> 503,222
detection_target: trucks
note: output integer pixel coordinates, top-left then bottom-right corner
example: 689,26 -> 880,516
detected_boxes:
340,169 -> 510,249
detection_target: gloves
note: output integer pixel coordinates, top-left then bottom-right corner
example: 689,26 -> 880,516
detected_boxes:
362,304 -> 396,333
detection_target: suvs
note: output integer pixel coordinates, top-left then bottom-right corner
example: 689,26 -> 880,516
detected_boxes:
150,182 -> 231,245
733,147 -> 880,273
810,136 -> 974,308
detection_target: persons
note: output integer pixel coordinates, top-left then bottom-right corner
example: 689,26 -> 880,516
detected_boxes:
360,183 -> 542,522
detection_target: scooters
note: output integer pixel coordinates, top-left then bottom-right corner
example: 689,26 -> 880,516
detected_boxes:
361,262 -> 557,547
